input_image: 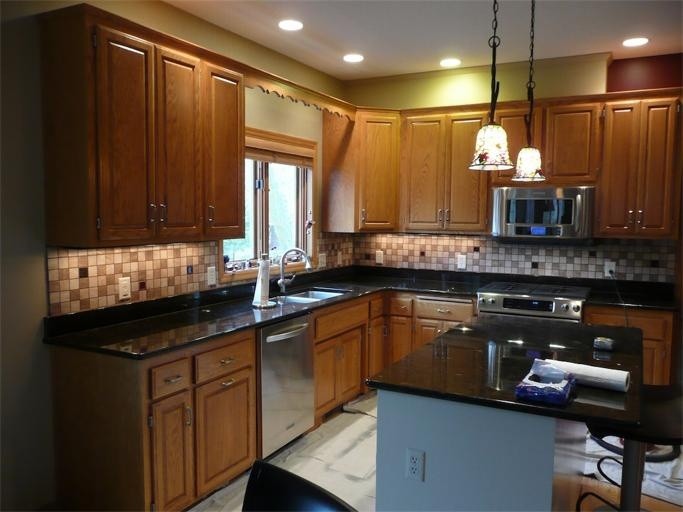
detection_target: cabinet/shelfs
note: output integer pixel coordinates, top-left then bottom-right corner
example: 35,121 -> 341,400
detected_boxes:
388,268 -> 425,366
321,106 -> 403,234
602,87 -> 683,239
55,311 -> 260,508
205,48 -> 248,238
584,278 -> 681,385
37,4 -> 204,248
492,94 -> 602,187
410,277 -> 478,351
337,277 -> 387,398
272,278 -> 368,422
402,102 -> 487,239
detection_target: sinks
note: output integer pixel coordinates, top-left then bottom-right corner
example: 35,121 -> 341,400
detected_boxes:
269,295 -> 320,303
290,290 -> 344,300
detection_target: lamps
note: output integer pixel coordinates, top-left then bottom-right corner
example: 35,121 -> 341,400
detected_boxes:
467,1 -> 513,174
512,1 -> 546,184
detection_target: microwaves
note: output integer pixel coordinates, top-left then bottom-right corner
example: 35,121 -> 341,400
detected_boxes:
490,186 -> 595,245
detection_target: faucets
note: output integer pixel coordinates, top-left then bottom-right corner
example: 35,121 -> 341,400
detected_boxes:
277,248 -> 313,292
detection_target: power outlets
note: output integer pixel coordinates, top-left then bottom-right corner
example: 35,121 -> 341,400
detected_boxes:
405,446 -> 425,481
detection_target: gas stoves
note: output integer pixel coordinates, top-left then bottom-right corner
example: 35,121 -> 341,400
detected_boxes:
476,277 -> 590,319
